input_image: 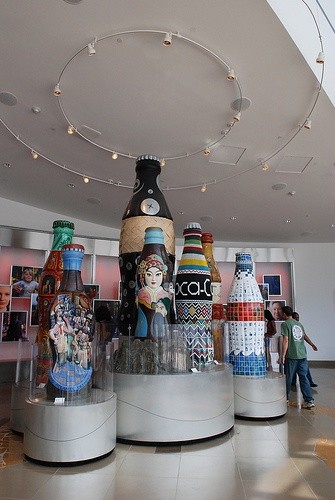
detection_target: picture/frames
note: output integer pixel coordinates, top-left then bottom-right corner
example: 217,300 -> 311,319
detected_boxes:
0,265 -> 122,342
257,273 -> 287,323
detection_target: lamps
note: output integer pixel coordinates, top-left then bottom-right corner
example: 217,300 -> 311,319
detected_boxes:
30,150 -> 38,159
200,184 -> 207,192
82,175 -> 89,184
162,32 -> 172,46
53,82 -> 62,97
227,69 -> 237,80
160,159 -> 165,166
204,147 -> 210,155
262,162 -> 268,170
233,111 -> 241,121
67,126 -> 76,135
304,118 -> 312,128
316,51 -> 326,63
87,39 -> 96,56
112,152 -> 117,159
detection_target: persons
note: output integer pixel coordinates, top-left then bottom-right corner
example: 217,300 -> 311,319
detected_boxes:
281,306 -> 318,409
0,286 -> 11,311
264,310 -> 277,371
12,269 -> 39,297
271,301 -> 282,321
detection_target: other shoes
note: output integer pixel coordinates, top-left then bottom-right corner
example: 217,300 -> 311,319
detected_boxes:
291,385 -> 297,392
310,384 -> 318,387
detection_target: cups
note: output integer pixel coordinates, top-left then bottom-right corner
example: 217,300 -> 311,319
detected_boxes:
46,242 -> 97,400
37,219 -> 80,385
201,230 -> 223,366
132,226 -> 175,361
228,253 -> 268,375
173,223 -> 215,369
119,154 -> 174,344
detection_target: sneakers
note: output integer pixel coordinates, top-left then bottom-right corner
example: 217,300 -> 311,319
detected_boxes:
301,400 -> 314,408
